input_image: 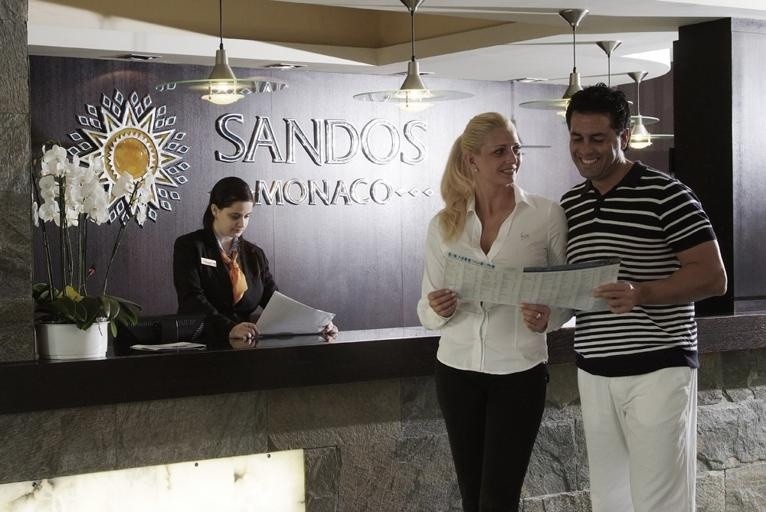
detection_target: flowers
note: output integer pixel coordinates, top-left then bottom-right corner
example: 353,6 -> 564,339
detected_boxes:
27,142 -> 155,339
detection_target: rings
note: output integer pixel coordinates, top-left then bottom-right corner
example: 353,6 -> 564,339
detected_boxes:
536,313 -> 541,320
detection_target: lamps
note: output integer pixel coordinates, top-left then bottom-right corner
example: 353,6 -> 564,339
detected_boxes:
355,0 -> 474,106
622,73 -> 660,150
519,8 -> 592,110
597,41 -> 622,91
167,1 -> 268,105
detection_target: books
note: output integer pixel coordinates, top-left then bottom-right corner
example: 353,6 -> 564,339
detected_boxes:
128,341 -> 208,352
255,290 -> 335,341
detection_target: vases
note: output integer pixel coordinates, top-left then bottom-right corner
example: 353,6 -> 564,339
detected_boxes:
37,320 -> 112,360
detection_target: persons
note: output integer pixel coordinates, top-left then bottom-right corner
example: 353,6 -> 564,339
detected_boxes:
560,83 -> 726,512
415,111 -> 568,512
173,176 -> 338,342
